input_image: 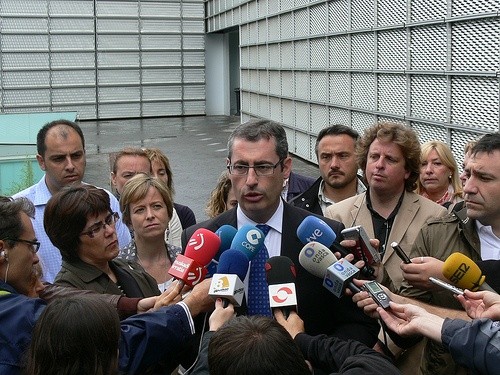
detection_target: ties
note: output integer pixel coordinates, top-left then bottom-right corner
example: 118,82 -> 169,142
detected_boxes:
246,225 -> 271,318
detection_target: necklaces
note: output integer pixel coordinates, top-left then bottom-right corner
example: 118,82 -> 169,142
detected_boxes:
137,248 -> 167,293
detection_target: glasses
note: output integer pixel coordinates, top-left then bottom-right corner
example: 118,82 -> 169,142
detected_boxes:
2,236 -> 41,253
227,156 -> 287,177
80,208 -> 120,237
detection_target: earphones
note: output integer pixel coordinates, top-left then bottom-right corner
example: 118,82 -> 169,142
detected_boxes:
1,251 -> 5,256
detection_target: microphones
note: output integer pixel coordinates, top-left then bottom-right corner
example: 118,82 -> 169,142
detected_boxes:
296,215 -> 376,301
168,224 -> 264,309
441,252 -> 497,295
264,256 -> 297,320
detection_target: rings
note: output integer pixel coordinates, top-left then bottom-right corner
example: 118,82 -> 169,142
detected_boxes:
397,322 -> 401,326
421,257 -> 424,263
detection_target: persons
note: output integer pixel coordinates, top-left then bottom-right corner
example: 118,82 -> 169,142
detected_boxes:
0,118 -> 500,375
